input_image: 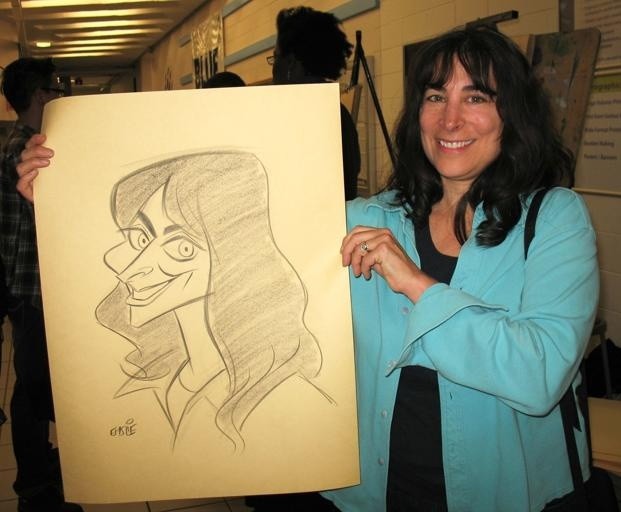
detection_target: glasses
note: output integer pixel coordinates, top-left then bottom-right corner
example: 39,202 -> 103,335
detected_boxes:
266,56 -> 275,65
41,86 -> 66,98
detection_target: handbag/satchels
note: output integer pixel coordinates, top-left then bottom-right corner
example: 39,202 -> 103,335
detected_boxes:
540,466 -> 619,511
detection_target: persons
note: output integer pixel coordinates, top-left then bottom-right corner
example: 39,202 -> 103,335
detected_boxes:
89,146 -> 345,457
262,4 -> 364,201
2,52 -> 86,511
12,25 -> 608,510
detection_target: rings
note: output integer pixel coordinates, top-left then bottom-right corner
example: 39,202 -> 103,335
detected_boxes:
361,241 -> 370,253
